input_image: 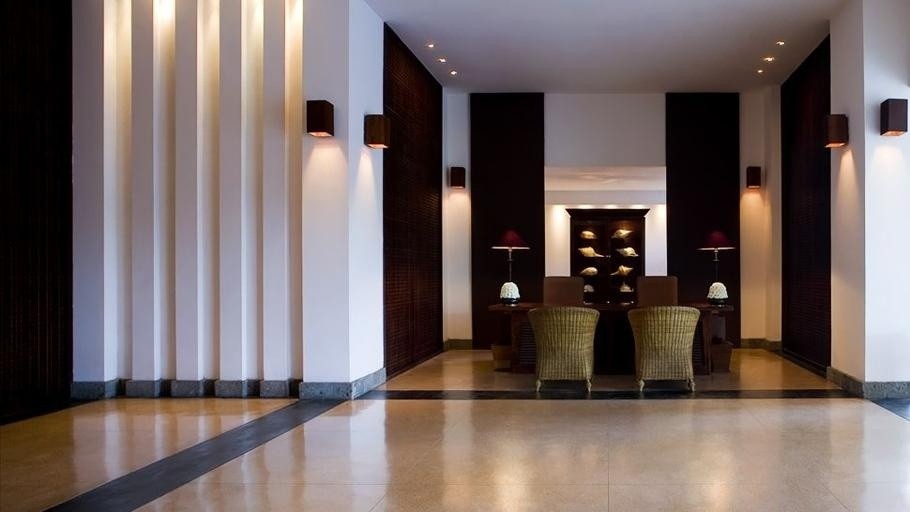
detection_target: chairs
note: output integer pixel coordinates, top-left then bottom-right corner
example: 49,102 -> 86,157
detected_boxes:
527,307 -> 600,393
629,305 -> 700,393
636,276 -> 679,307
543,276 -> 584,307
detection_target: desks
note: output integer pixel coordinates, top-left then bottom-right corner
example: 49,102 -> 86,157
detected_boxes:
492,302 -> 735,375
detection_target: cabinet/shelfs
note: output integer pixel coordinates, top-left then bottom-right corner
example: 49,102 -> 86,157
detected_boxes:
566,209 -> 650,305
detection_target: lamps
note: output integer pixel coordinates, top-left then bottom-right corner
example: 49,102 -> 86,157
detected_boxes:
823,114 -> 848,148
363,114 -> 391,150
879,99 -> 907,137
451,166 -> 467,190
699,232 -> 736,307
746,165 -> 761,189
306,99 -> 335,137
493,231 -> 532,305
